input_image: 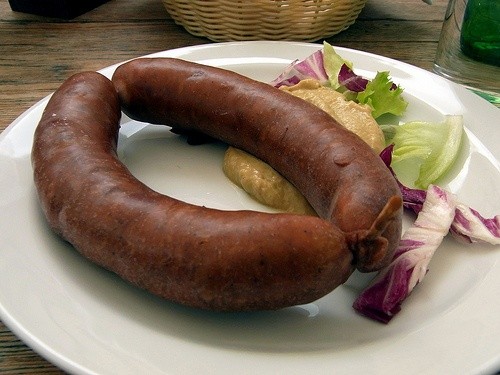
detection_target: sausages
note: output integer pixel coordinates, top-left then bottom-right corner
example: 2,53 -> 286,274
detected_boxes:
113,56 -> 404,270
22,70 -> 355,314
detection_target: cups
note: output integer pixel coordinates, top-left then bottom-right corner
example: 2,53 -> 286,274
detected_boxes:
435,0 -> 500,106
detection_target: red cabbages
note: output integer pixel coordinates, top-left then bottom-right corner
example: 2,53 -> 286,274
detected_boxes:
270,50 -> 500,323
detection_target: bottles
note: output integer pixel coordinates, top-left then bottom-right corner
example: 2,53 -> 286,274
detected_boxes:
460,0 -> 500,67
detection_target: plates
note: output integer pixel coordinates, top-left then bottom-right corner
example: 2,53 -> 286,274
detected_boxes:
2,38 -> 499,374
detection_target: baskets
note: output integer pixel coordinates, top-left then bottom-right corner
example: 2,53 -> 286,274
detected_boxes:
161,0 -> 367,43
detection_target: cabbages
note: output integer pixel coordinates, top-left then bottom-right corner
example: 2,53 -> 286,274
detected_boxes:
322,39 -> 469,187
